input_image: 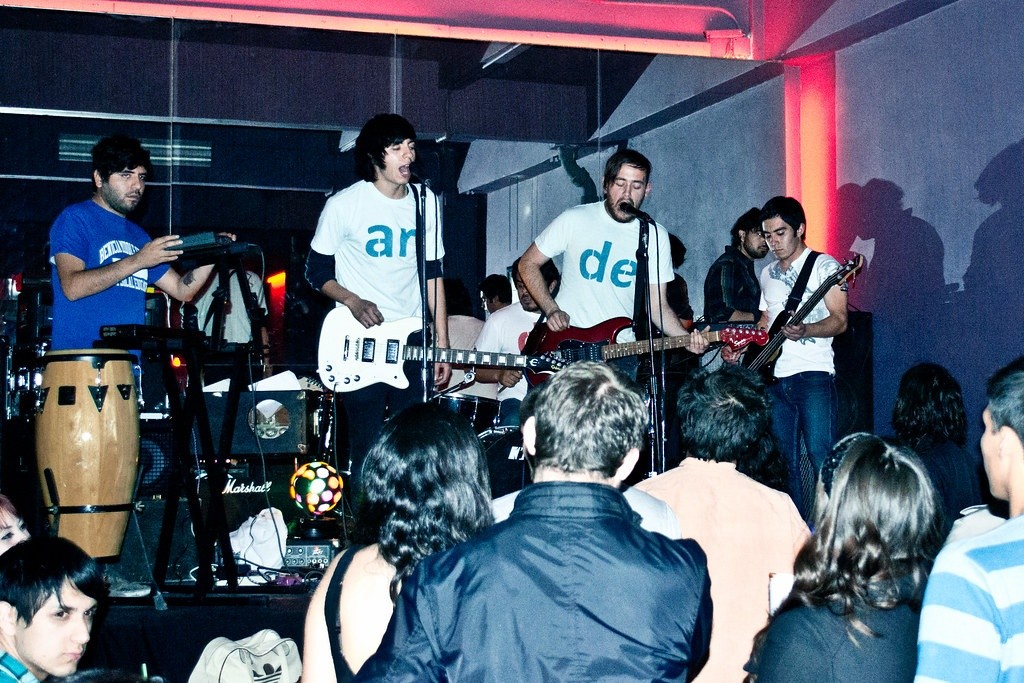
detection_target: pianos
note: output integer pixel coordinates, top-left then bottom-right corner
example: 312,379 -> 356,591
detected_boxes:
656,321 -> 756,339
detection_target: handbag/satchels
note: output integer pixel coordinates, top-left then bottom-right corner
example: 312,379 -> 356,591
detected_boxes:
189,627 -> 302,683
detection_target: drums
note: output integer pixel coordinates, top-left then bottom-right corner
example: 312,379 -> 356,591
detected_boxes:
32,346 -> 142,560
307,392 -> 335,468
444,391 -> 502,439
476,428 -> 506,450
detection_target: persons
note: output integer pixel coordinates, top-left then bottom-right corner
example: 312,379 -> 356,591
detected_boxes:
0,494 -> 32,555
303,352 -> 1024,683
304,112 -> 452,508
180,228 -> 274,391
50,133 -> 236,363
446,251 -> 562,435
517,148 -> 709,401
704,206 -> 769,345
645,232 -> 694,458
746,196 -> 849,521
0,531 -> 103,683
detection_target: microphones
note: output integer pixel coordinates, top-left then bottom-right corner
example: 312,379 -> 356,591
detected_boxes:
409,161 -> 443,196
620,202 -> 656,227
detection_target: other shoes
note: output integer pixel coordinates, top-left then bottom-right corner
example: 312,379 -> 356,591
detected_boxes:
100,570 -> 151,597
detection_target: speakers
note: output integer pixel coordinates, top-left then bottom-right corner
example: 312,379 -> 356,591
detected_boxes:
798,311 -> 875,527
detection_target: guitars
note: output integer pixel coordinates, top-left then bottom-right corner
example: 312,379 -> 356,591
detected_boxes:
744,251 -> 865,395
316,304 -> 542,394
522,316 -> 771,388
